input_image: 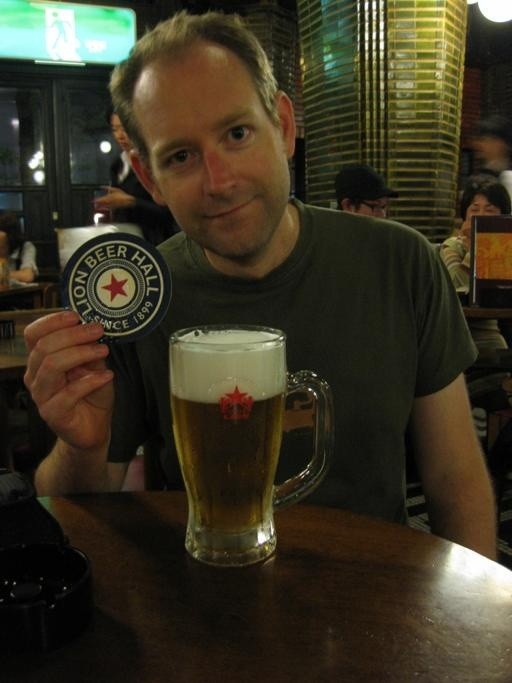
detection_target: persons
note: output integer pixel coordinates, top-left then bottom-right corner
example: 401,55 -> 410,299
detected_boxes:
19,7 -> 502,564
91,104 -> 183,247
433,174 -> 511,308
332,162 -> 398,220
466,112 -> 511,199
0,208 -> 42,287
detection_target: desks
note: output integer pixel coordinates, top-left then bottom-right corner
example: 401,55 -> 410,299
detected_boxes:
1,493 -> 512,680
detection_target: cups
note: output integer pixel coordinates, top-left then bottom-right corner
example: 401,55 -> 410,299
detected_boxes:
163,320 -> 335,576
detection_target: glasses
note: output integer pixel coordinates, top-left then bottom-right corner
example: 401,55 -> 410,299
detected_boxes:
359,201 -> 386,212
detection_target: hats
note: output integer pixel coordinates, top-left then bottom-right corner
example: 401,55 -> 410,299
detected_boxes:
335,164 -> 399,200
